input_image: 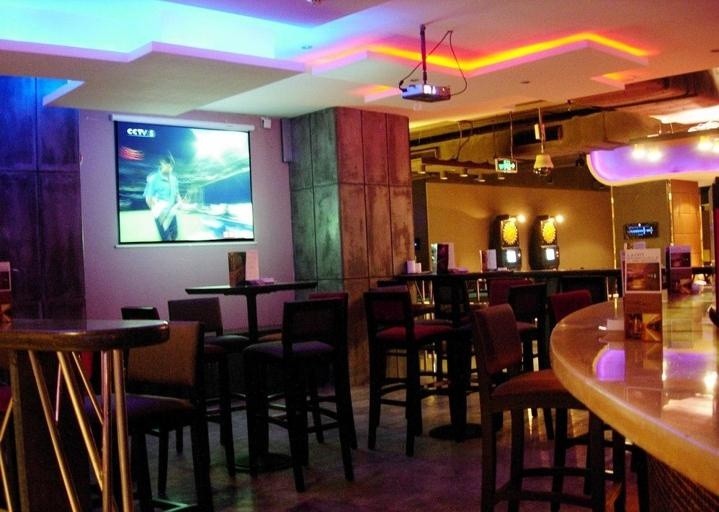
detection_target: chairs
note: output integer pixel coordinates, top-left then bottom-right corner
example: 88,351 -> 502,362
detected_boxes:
260,292 -> 349,442
245,297 -> 354,489
370,273 -> 622,437
473,304 -> 628,511
82,321 -> 216,510
119,298 -> 243,459
362,292 -> 453,454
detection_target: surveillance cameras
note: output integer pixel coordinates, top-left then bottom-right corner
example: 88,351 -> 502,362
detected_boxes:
533,154 -> 555,177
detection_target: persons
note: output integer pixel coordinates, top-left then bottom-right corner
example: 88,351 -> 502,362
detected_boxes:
144,151 -> 196,241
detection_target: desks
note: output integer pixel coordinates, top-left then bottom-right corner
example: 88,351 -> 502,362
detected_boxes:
0,318 -> 171,512
185,282 -> 318,451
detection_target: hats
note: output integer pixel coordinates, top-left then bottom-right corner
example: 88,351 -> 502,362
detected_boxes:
159,150 -> 175,163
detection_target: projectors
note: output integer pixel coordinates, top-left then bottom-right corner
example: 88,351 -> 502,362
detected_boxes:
401,81 -> 453,103
